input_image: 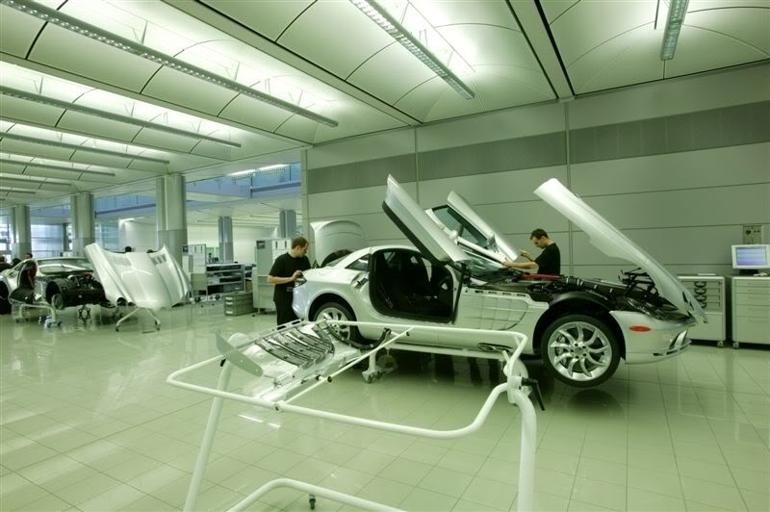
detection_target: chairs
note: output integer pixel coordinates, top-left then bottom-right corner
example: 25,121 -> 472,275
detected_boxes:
371,252 -> 450,317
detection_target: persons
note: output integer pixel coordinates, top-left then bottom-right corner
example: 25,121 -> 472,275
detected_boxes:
12,258 -> 22,269
23,254 -> 37,279
266,237 -> 311,330
502,227 -> 560,277
0,256 -> 13,315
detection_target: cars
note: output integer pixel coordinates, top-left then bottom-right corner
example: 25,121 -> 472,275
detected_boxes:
0,240 -> 195,315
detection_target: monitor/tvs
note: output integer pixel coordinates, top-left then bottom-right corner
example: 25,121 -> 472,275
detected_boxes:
731,244 -> 770,275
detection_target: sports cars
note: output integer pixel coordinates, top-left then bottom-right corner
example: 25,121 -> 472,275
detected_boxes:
287,170 -> 711,387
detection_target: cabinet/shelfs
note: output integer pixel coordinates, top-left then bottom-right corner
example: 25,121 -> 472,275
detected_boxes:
723,273 -> 770,345
183,237 -> 293,317
